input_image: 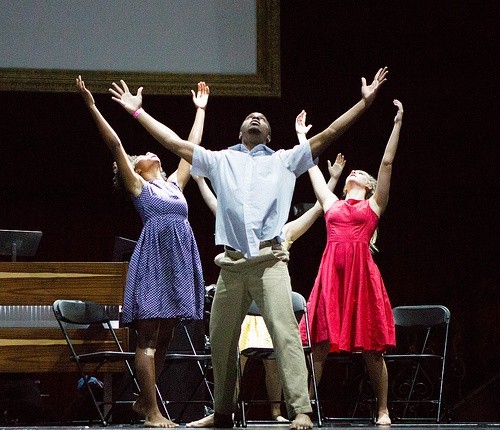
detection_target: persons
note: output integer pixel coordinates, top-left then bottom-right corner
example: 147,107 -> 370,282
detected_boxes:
191,153 -> 347,422
295,100 -> 403,426
109,66 -> 388,429
76,75 -> 210,428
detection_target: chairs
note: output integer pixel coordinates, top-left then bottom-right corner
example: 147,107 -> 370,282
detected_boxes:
52,291 -> 451,427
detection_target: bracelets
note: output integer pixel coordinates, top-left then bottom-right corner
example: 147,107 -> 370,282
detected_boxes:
133,107 -> 144,118
